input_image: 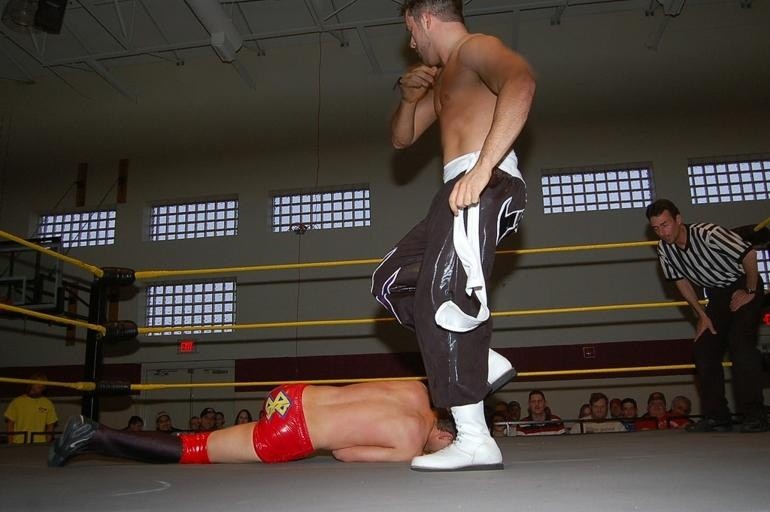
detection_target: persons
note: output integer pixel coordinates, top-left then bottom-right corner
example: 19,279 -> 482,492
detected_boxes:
370,0 -> 537,473
3,372 -> 59,446
123,408 -> 265,433
438,391 -> 696,437
646,199 -> 769,434
48,380 -> 457,468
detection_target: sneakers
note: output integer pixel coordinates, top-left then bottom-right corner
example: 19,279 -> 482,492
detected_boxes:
484,348 -> 518,393
46,412 -> 99,469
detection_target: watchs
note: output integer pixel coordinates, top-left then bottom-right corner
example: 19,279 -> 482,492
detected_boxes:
744,287 -> 756,294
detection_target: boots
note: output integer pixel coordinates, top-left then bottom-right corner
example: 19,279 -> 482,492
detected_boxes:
409,398 -> 507,473
